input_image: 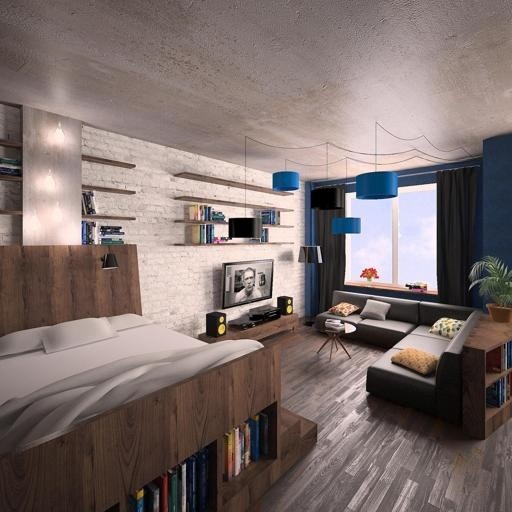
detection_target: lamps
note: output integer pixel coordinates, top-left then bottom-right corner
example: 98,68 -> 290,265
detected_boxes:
228,121 -> 399,238
298,246 -> 324,326
100,246 -> 118,270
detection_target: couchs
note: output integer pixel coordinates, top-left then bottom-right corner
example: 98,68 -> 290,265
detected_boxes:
315,289 -> 484,426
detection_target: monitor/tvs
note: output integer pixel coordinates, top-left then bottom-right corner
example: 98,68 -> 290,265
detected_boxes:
220,259 -> 274,309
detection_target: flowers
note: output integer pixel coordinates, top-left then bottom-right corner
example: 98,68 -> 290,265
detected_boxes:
360,268 -> 380,285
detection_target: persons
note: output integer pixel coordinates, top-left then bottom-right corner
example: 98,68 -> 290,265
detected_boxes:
234,267 -> 263,302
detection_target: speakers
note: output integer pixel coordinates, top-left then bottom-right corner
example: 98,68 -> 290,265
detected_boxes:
206,312 -> 227,337
277,296 -> 294,315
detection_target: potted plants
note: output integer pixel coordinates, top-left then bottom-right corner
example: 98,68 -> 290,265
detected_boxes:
469,255 -> 512,322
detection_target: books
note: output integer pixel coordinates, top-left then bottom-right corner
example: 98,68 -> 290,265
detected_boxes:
188,203 -> 231,244
133,412 -> 271,511
80,188 -> 128,245
487,340 -> 512,408
1,155 -> 23,176
260,208 -> 282,241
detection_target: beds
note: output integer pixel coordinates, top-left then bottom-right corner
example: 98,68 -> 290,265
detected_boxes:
0,244 -> 317,512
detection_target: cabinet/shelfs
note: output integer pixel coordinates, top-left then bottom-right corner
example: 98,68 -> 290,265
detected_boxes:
0,137 -> 137,221
462,313 -> 512,440
174,172 -> 295,229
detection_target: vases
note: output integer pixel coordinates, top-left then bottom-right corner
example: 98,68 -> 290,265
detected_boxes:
366,279 -> 372,287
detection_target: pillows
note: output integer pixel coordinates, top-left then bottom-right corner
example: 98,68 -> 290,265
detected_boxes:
328,298 -> 391,321
428,316 -> 467,340
0,313 -> 154,357
391,346 -> 440,376
